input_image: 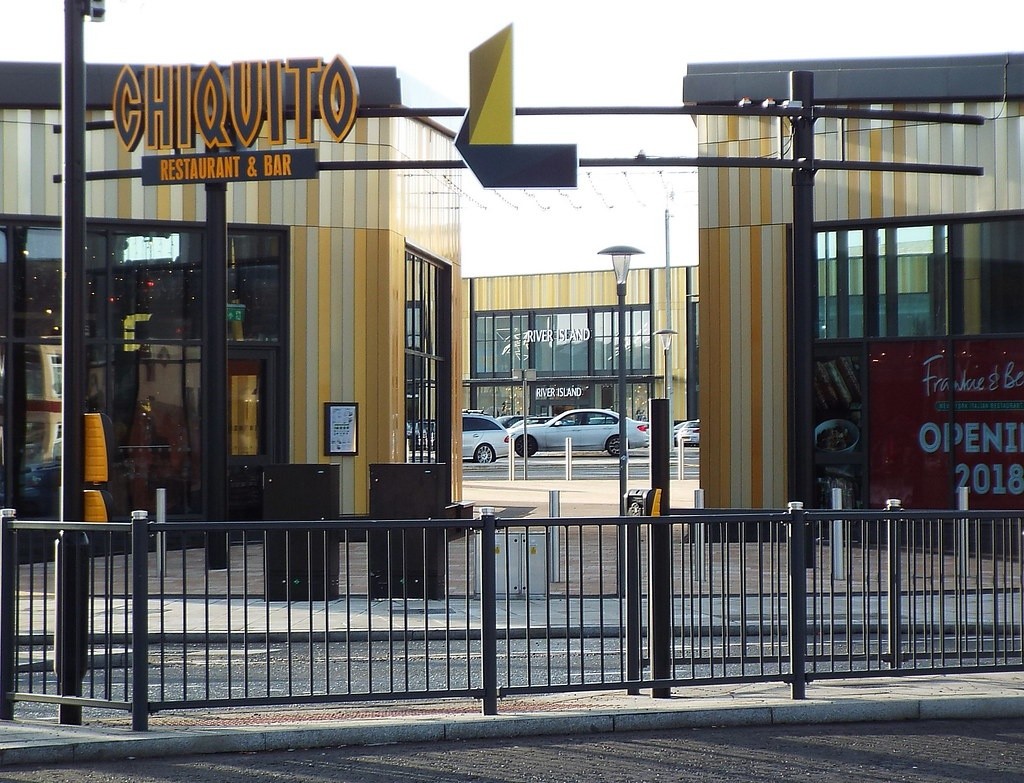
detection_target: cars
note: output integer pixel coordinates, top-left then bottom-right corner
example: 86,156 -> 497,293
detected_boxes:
495,415 -> 616,431
462,408 -> 511,464
673,419 -> 700,447
510,409 -> 649,458
406,421 -> 437,452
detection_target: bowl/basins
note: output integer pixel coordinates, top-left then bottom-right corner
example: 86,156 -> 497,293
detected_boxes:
815,419 -> 860,452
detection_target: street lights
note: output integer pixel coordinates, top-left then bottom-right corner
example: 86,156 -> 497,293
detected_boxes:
58,0 -> 105,726
652,329 -> 679,399
597,245 -> 645,599
